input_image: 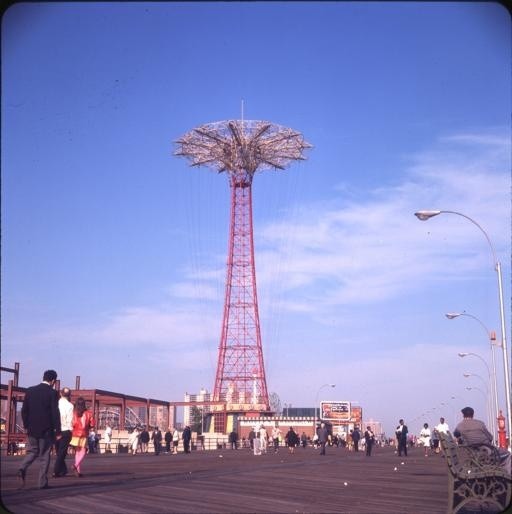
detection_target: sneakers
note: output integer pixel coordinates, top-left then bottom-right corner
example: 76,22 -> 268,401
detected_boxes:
52,472 -> 68,478
40,486 -> 51,489
17,468 -> 25,488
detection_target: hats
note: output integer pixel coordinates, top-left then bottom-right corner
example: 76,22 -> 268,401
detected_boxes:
461,407 -> 474,415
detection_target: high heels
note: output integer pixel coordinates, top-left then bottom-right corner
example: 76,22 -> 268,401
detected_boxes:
72,464 -> 81,477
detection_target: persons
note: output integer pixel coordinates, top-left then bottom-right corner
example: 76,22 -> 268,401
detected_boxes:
70,397 -> 95,476
16,370 -> 61,490
455,407 -> 507,472
90,421 -> 192,456
229,417 -> 449,456
51,387 -> 75,478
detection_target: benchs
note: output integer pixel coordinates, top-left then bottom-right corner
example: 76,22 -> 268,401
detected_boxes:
437,428 -> 512,514
453,430 -> 503,468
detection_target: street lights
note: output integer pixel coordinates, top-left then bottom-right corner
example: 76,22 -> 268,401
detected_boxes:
314,383 -> 337,434
413,352 -> 498,450
413,206 -> 512,450
446,310 -> 500,448
